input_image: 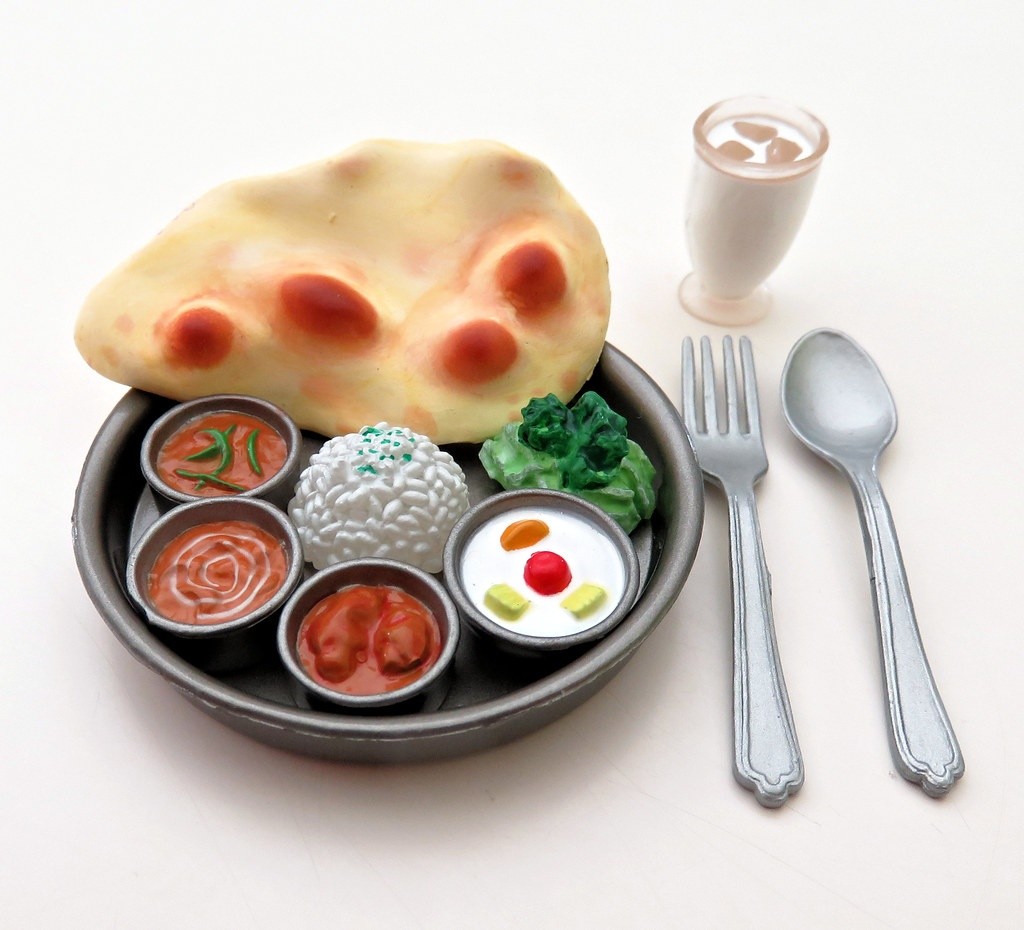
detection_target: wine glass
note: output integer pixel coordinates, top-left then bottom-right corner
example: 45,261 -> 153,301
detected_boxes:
679,95 -> 829,325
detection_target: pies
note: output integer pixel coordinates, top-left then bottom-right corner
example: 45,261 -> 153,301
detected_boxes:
71,137 -> 612,446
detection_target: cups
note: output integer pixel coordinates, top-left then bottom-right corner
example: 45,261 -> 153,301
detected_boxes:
443,487 -> 639,651
277,558 -> 457,713
142,394 -> 301,515
126,497 -> 305,650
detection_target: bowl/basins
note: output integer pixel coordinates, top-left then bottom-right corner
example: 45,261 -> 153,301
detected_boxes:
72,345 -> 702,765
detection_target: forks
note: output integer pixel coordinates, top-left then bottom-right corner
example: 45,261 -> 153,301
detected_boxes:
682,335 -> 804,808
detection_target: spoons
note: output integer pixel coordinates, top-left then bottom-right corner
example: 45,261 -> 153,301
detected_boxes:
781,329 -> 964,800
